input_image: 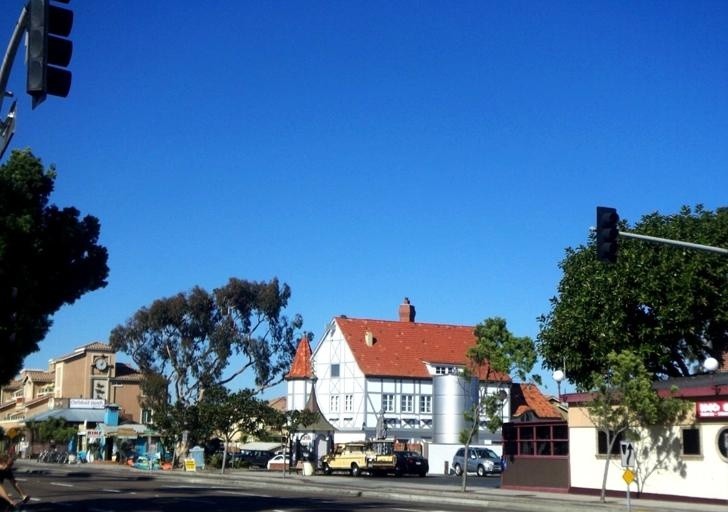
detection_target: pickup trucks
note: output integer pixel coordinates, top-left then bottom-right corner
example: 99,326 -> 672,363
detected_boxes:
319,442 -> 398,477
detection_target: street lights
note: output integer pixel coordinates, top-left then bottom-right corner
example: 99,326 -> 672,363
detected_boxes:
704,357 -> 719,374
553,370 -> 564,398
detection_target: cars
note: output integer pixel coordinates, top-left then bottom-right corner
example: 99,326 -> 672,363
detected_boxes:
452,447 -> 502,477
369,450 -> 429,478
267,455 -> 290,471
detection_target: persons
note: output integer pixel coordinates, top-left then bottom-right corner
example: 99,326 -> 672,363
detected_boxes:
0,426 -> 31,512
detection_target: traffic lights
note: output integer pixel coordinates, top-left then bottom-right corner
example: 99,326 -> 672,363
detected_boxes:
27,1 -> 74,110
596,205 -> 620,264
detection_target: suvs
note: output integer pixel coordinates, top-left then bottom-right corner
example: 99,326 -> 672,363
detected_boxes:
227,449 -> 276,468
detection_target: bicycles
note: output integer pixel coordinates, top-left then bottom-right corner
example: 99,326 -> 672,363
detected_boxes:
36,448 -> 70,464
